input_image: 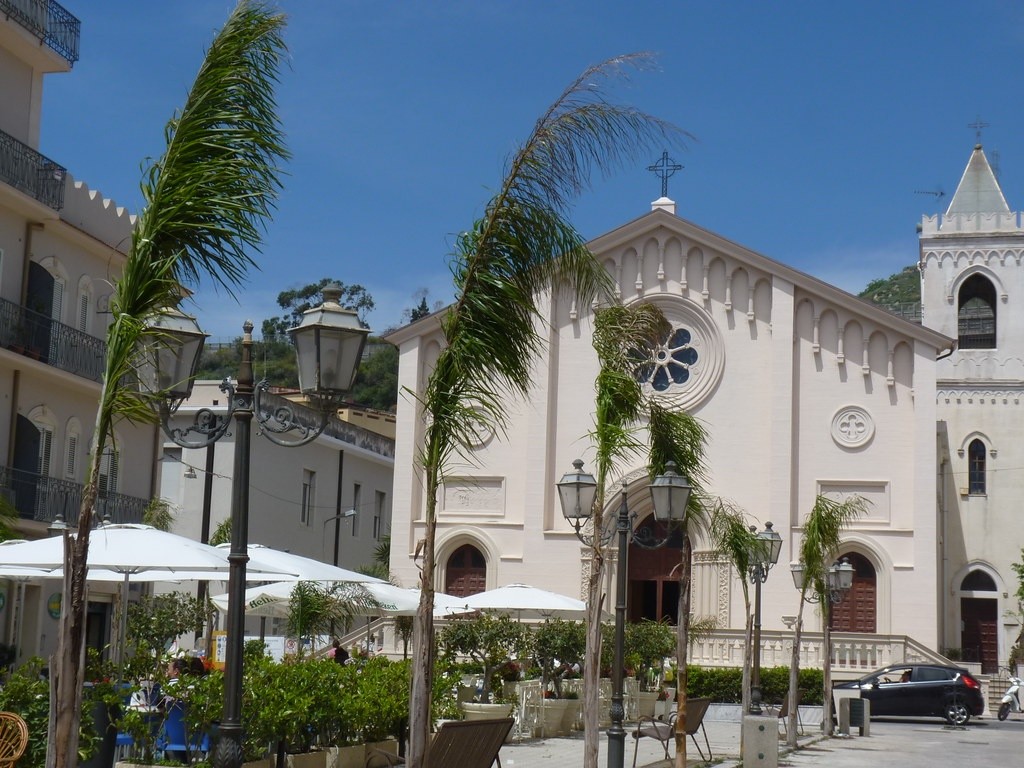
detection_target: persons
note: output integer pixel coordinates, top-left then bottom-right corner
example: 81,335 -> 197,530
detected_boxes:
897,671 -> 912,683
156,659 -> 200,707
491,657 -> 639,690
332,639 -> 349,668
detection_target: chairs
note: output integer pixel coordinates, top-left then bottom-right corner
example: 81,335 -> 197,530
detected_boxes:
0,711 -> 29,768
632,697 -> 713,768
765,688 -> 804,741
363,717 -> 515,768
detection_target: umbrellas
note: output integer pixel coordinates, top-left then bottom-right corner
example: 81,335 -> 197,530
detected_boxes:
0,524 -> 299,691
207,543 -> 618,641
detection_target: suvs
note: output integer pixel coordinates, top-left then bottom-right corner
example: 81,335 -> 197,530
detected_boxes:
831,662 -> 985,725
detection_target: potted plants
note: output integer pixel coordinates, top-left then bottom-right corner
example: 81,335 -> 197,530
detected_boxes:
186,612 -> 677,768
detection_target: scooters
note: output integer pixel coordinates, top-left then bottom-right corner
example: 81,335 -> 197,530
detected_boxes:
997,675 -> 1024,721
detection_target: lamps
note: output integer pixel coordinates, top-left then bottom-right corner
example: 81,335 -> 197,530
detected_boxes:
324,510 -> 357,524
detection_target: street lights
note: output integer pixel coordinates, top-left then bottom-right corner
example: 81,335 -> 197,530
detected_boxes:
555,459 -> 696,768
748,522 -> 783,714
789,556 -> 856,651
132,283 -> 374,768
